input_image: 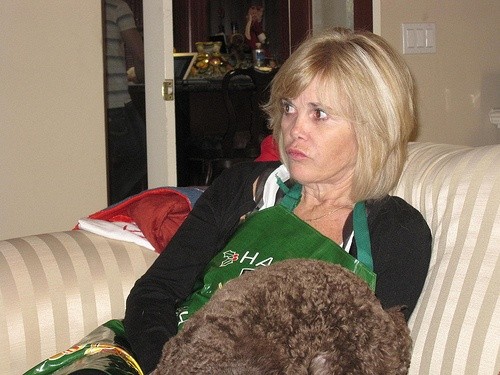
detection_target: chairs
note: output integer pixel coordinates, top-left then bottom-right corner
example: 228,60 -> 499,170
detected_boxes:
187,68 -> 259,185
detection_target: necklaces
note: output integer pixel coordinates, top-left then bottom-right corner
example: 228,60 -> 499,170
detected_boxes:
299,204 -> 350,222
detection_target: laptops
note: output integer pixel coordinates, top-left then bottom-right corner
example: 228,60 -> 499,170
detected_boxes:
174,52 -> 198,82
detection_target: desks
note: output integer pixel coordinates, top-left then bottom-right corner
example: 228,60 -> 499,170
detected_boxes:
127,78 -> 269,146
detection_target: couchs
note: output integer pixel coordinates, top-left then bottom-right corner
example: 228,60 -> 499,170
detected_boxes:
0,141 -> 500,375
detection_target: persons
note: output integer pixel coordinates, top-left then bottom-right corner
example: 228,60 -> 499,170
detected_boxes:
105,0 -> 148,205
23,25 -> 433,375
245,6 -> 273,65
